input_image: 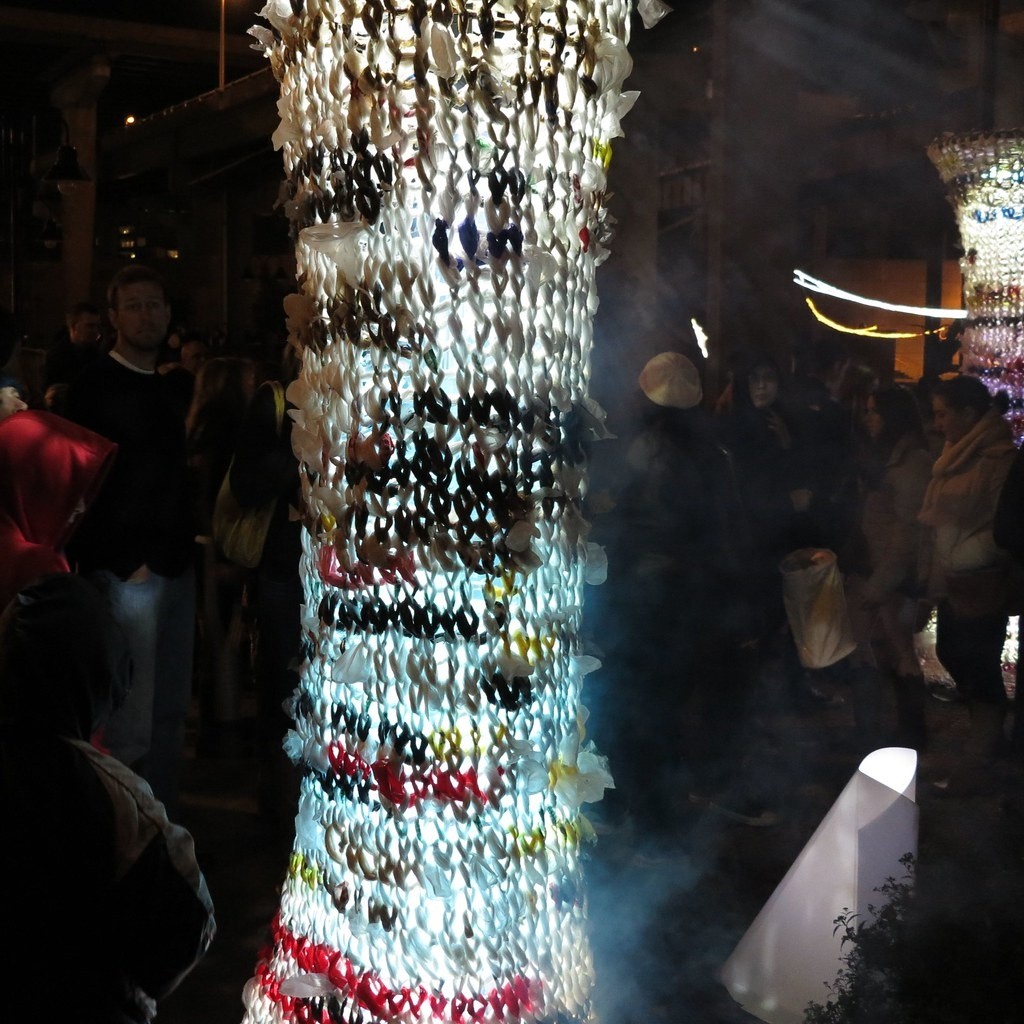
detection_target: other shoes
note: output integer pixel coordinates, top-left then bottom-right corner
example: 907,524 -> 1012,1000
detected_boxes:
688,781 -> 712,805
710,787 -> 778,826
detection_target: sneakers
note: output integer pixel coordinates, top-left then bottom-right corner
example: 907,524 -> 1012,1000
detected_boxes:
782,682 -> 844,710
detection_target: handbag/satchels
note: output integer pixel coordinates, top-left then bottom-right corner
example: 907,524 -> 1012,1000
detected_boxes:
210,380 -> 285,570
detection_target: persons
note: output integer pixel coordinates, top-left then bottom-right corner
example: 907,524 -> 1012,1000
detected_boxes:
918,375 -> 1023,768
995,442 -> 1024,565
1,578 -> 218,1022
851,390 -> 926,748
583,350 -> 850,867
0,268 -> 297,845
